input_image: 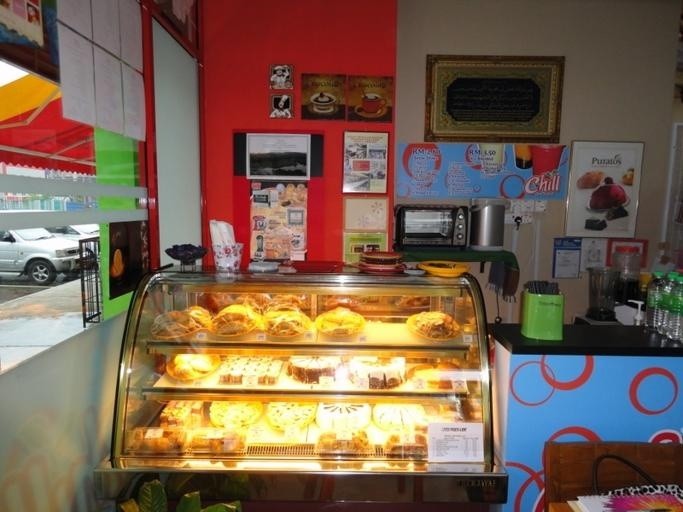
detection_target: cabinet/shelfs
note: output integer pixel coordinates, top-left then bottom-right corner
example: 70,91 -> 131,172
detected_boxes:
91,263 -> 508,510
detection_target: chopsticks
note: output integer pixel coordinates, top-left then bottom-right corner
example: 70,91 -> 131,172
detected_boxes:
527,280 -> 558,294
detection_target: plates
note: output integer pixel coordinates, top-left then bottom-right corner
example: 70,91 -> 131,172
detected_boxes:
354,104 -> 388,119
352,250 -> 471,278
308,103 -> 339,114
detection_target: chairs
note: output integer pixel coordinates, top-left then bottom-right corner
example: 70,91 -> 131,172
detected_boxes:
544,441 -> 683,512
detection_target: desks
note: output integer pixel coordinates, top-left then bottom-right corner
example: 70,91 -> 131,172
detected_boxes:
549,503 -> 574,512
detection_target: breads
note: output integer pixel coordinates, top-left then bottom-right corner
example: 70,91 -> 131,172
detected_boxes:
167,352 -> 215,378
198,292 -> 359,316
461,399 -> 482,421
415,311 -> 454,338
398,296 -> 431,306
151,302 -> 363,339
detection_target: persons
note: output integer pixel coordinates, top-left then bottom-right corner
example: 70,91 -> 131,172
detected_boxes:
270,65 -> 291,118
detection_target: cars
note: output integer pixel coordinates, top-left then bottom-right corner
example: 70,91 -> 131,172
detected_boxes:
44,225 -> 101,274
0,228 -> 90,286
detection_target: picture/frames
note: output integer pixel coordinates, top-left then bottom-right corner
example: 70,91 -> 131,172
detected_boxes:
341,130 -> 390,194
343,196 -> 388,262
269,95 -> 294,120
563,140 -> 645,239
268,64 -> 293,91
607,239 -> 648,268
246,133 -> 311,181
425,53 -> 565,144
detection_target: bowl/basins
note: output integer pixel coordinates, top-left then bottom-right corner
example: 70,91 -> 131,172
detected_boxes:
164,249 -> 208,264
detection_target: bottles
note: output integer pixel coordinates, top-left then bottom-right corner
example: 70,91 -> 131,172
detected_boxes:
645,268 -> 682,341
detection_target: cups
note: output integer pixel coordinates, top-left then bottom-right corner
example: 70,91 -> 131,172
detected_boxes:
310,92 -> 337,113
359,93 -> 387,112
585,252 -> 640,319
213,243 -> 243,275
514,143 -> 567,177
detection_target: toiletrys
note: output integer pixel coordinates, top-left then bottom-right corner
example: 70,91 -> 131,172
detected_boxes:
627,298 -> 645,326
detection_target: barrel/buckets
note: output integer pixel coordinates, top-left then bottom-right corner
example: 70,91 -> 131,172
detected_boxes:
471,200 -> 507,248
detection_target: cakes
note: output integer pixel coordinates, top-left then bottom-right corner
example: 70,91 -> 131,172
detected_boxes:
124,401 -> 431,467
221,355 -> 459,390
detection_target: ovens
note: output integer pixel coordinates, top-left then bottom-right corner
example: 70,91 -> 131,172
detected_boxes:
395,204 -> 469,247
